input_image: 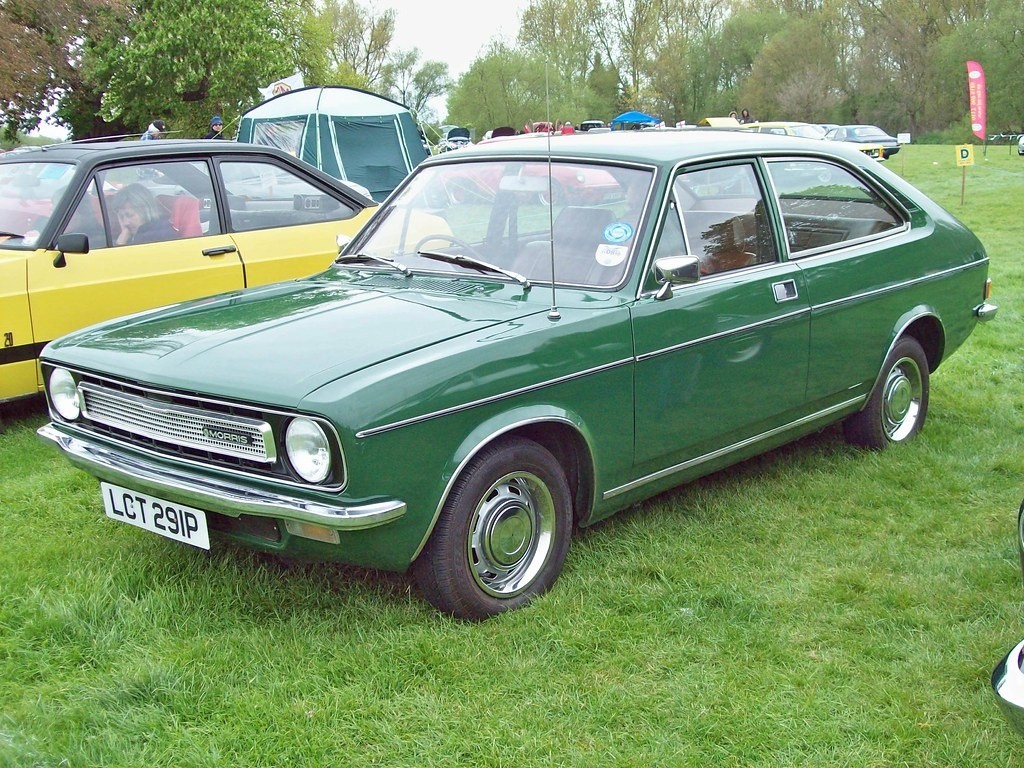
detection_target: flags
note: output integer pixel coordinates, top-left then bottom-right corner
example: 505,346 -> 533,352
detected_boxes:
258,72 -> 307,102
965,60 -> 987,140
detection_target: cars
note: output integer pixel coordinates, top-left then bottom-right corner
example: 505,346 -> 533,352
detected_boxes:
445,121 -> 603,152
1,146 -> 458,411
700,117 -> 901,161
1017,135 -> 1024,154
439,159 -> 622,208
35,129 -> 998,618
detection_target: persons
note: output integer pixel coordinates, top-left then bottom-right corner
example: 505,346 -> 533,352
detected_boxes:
729,110 -> 738,120
109,182 -> 183,247
560,121 -> 576,134
739,108 -> 755,124
204,116 -> 226,140
141,120 -> 165,140
51,185 -> 109,250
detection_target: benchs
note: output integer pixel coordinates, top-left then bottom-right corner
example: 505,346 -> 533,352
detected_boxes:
683,210 -> 893,261
200,210 -> 326,235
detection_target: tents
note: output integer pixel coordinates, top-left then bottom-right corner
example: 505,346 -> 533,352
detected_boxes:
608,110 -> 661,130
237,84 -> 430,206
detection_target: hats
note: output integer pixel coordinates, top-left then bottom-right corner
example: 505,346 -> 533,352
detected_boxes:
153,120 -> 166,132
211,117 -> 223,126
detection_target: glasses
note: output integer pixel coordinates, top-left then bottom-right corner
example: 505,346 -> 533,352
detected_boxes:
214,123 -> 222,126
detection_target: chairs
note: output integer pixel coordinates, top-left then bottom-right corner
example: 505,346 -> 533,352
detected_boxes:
156,195 -> 203,238
509,205 -> 616,286
90,191 -> 121,237
694,214 -> 757,275
